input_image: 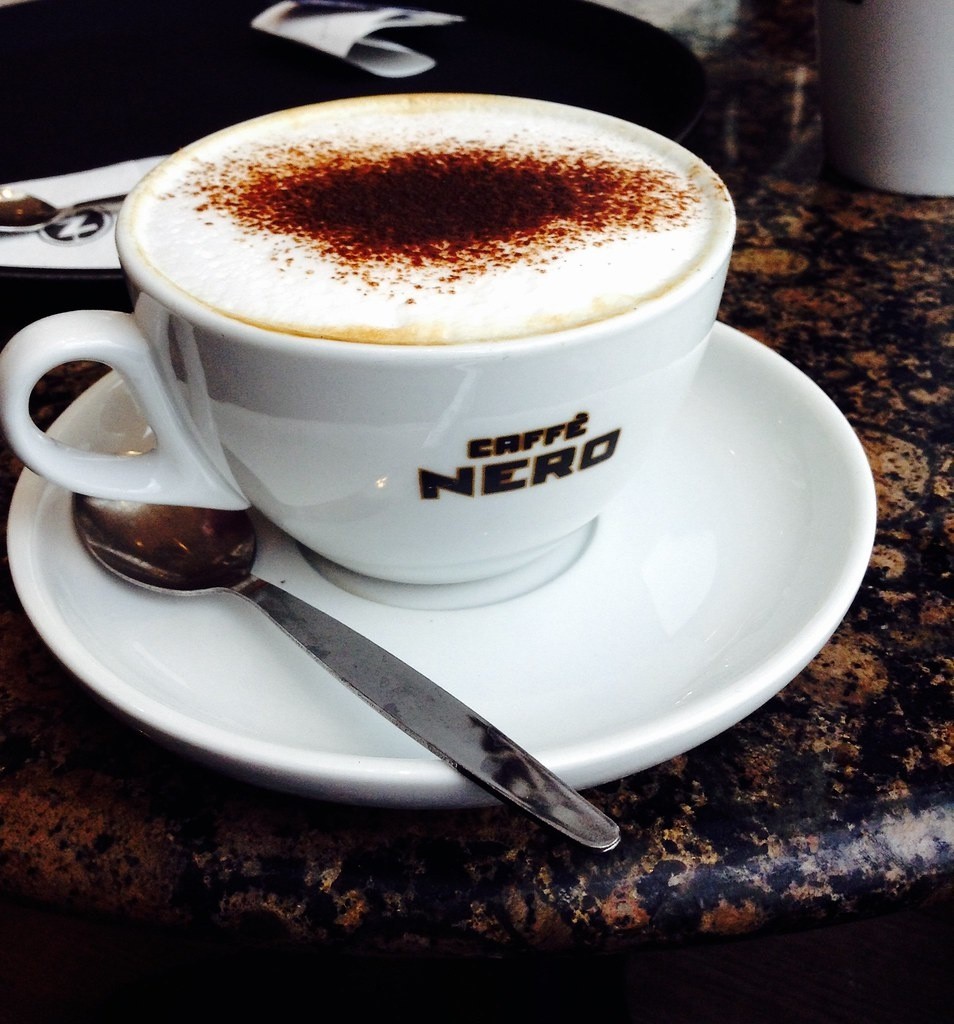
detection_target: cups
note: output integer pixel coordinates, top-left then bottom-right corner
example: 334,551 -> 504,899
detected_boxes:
0,92 -> 739,584
817,0 -> 954,200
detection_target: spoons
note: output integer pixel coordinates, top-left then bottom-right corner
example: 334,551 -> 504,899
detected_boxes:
0,186 -> 127,235
71,448 -> 623,857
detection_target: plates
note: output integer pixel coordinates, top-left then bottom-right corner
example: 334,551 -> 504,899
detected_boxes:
7,317 -> 876,802
